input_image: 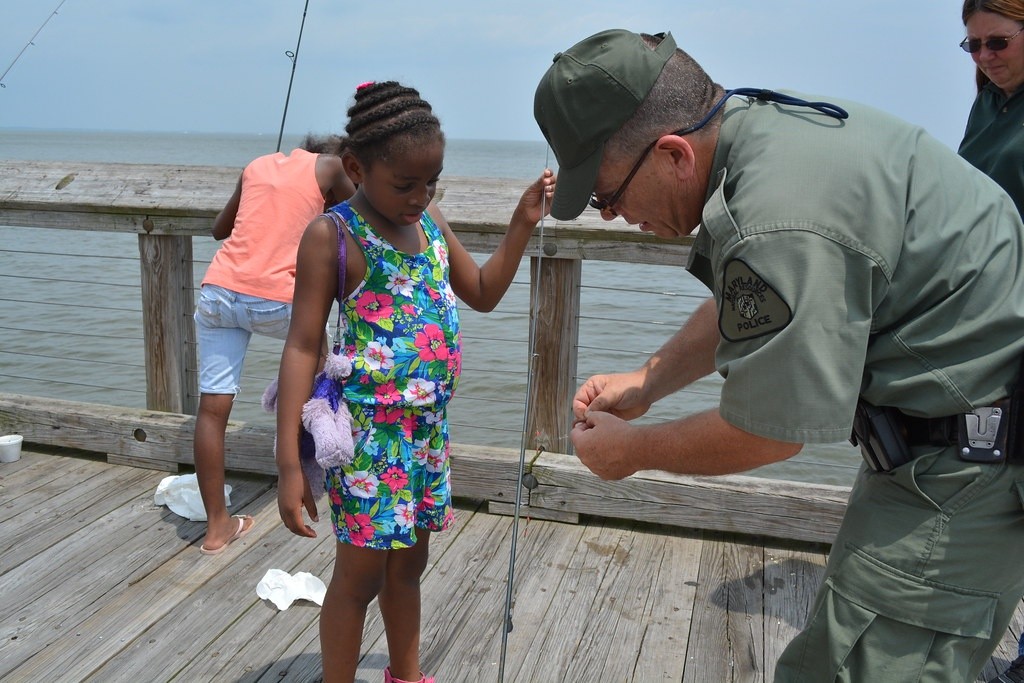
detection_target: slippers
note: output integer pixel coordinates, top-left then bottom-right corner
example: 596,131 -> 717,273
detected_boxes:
202,514 -> 256,553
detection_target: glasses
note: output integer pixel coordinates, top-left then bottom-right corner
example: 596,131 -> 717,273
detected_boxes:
960,26 -> 1024,53
588,126 -> 675,217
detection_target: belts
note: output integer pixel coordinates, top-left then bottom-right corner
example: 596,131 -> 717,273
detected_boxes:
891,400 -> 1020,460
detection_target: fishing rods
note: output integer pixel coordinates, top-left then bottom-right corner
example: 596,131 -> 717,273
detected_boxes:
498,141 -> 550,683
0,0 -> 66,80
276,0 -> 309,153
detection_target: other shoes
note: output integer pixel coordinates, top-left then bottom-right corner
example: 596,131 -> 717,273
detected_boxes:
381,666 -> 435,683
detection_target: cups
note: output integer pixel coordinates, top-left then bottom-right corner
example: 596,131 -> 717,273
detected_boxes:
0,434 -> 25,463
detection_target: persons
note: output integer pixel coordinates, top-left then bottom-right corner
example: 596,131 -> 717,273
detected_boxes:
959,0 -> 1024,222
274,80 -> 558,683
192,135 -> 357,555
534,28 -> 1024,682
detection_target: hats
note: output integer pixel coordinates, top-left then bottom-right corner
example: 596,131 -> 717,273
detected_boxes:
533,28 -> 677,221
